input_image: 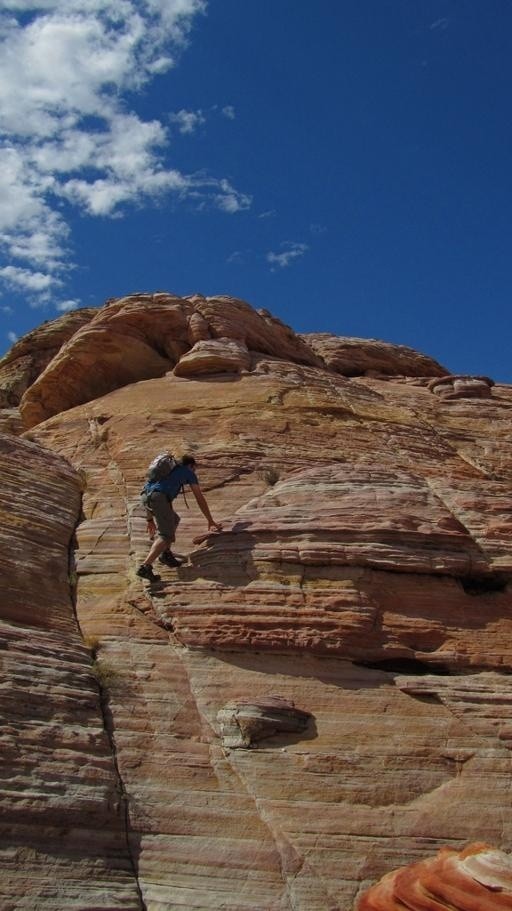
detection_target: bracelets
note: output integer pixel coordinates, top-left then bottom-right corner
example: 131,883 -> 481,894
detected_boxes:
147,518 -> 153,523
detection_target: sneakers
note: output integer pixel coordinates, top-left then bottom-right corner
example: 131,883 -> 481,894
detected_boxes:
136,564 -> 160,582
159,551 -> 180,567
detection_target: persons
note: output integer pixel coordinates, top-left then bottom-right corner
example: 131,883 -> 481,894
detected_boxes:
133,451 -> 224,581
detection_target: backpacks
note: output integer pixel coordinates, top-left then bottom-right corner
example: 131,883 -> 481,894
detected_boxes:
146,451 -> 177,484
